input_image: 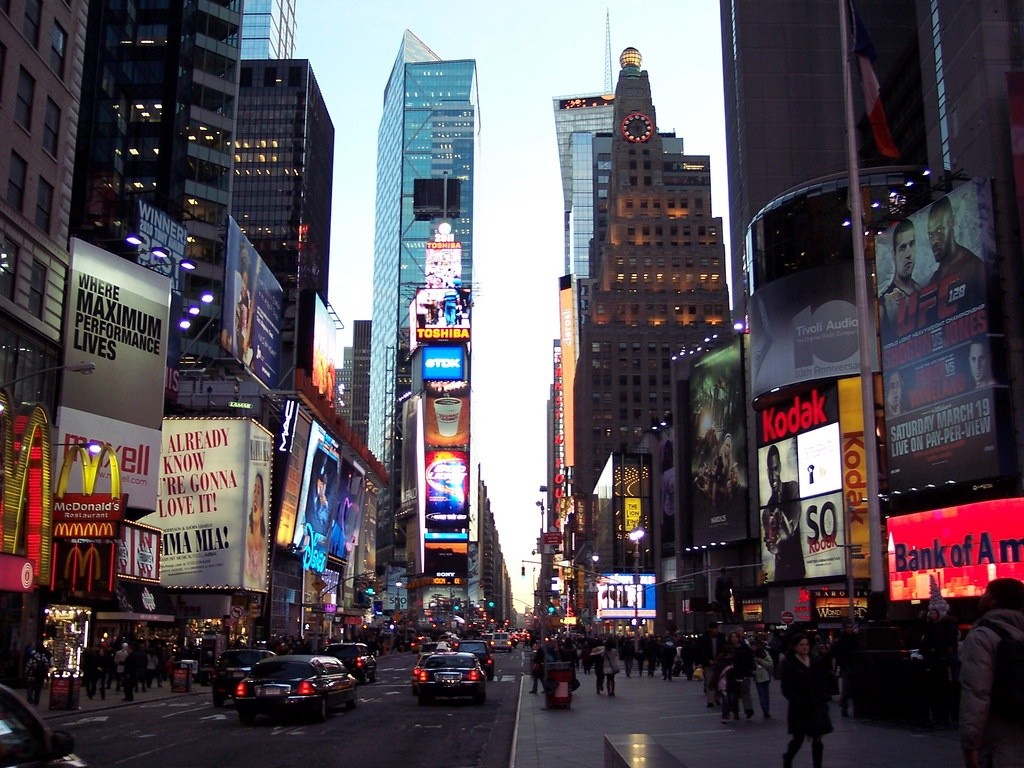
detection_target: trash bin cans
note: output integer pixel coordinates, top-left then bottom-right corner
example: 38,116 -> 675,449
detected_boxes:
49,669 -> 80,710
540,662 -> 573,708
171,661 -> 193,692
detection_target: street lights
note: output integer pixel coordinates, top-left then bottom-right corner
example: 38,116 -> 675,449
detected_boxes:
631,526 -> 648,648
395,578 -> 403,613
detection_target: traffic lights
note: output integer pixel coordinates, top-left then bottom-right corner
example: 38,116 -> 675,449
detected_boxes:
366,587 -> 374,595
489,602 -> 495,608
454,597 -> 460,610
547,606 -> 555,614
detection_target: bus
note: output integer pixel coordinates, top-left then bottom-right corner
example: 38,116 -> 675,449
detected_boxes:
411,652 -> 434,697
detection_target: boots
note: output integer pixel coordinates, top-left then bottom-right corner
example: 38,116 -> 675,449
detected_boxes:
782,740 -> 800,768
812,741 -> 823,768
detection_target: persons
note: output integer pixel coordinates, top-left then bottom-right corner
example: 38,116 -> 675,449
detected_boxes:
958,579 -> 1024,768
393,627 -> 481,651
523,635 -> 561,694
416,249 -> 470,327
877,197 -> 983,347
762,445 -> 806,581
236,235 -> 254,364
816,621 -> 856,717
247,474 -> 267,588
233,636 -> 258,648
710,433 -> 732,510
562,621 -> 786,723
779,635 -> 834,768
320,631 -> 385,656
885,368 -> 906,419
299,475 -> 344,569
80,641 -> 199,702
24,642 -> 53,706
970,335 -> 994,389
920,611 -> 956,671
267,633 -> 313,655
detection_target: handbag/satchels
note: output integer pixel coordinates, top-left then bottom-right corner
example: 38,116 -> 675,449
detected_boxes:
532,663 -> 540,672
610,660 -> 620,673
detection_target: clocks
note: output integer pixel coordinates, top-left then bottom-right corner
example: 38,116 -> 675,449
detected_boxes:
622,114 -> 654,143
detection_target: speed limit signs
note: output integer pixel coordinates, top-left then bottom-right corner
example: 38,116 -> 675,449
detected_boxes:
781,611 -> 795,625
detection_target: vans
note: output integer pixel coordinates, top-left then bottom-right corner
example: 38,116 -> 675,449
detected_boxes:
456,639 -> 495,681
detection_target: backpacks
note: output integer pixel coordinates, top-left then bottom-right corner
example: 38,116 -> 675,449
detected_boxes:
33,656 -> 46,680
975,618 -> 1024,727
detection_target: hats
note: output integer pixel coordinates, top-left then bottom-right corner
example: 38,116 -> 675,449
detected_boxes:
989,579 -> 1024,610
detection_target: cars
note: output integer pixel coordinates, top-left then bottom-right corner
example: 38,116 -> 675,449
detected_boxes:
483,630 -> 531,652
322,642 -> 376,685
411,633 -> 459,663
416,653 -> 487,706
0,683 -> 95,768
211,650 -> 278,708
232,654 -> 357,724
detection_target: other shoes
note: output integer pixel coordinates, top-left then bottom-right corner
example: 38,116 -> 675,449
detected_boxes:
747,710 -> 752,718
529,690 -> 536,694
722,718 -> 726,723
716,702 -> 720,705
764,712 -> 770,718
726,713 -> 728,720
708,703 -> 713,707
736,715 -> 739,720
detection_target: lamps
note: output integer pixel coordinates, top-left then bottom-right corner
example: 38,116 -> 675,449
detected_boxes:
145,259 -> 199,270
50,441 -> 104,453
0,361 -> 96,390
93,234 -> 144,243
115,246 -> 171,256
170,287 -> 213,328
